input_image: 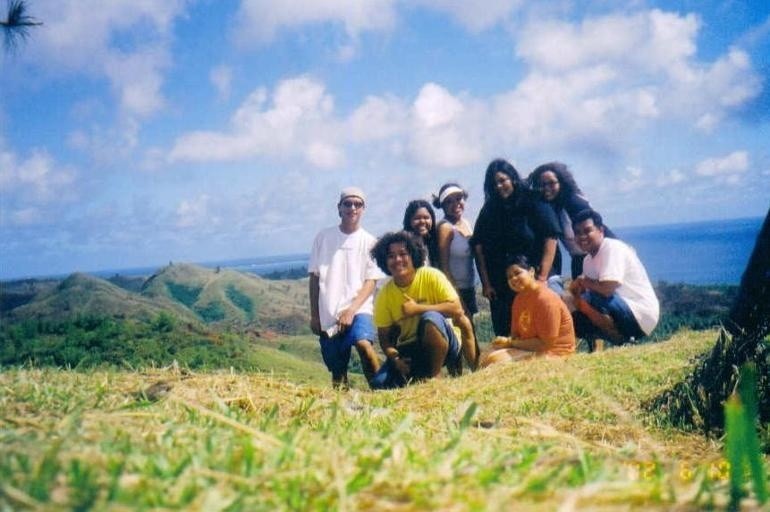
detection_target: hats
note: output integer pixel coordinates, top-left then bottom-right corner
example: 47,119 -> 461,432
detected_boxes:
340,188 -> 365,206
439,184 -> 463,205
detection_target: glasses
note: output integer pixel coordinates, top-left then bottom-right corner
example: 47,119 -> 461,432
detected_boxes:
343,201 -> 363,208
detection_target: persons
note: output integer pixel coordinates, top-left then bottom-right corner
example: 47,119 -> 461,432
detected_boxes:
308,187 -> 386,392
402,200 -> 441,270
431,182 -> 481,374
524,160 -> 618,353
569,207 -> 661,352
483,253 -> 576,367
368,230 -> 465,388
469,158 -> 563,337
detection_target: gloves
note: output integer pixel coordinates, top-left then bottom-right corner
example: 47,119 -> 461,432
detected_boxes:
388,353 -> 411,377
